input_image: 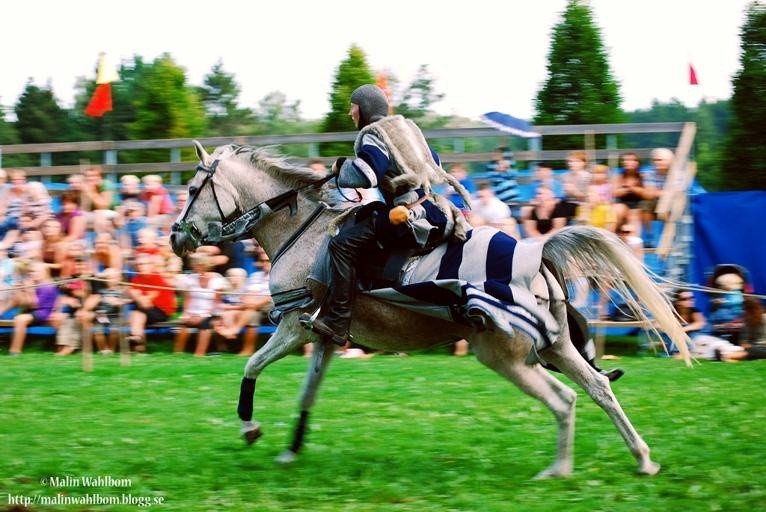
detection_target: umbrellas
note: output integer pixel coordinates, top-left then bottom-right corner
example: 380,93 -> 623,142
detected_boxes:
479,112 -> 543,147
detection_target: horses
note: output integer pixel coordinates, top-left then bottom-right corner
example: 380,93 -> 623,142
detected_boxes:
167,139 -> 699,482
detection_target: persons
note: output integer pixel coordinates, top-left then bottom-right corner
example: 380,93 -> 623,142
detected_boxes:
0,148 -> 766,362
298,81 -> 473,347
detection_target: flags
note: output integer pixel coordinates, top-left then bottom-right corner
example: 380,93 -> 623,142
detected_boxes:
84,84 -> 113,118
95,53 -> 122,85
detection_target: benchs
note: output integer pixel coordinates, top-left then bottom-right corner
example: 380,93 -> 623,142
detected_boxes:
1,161 -> 696,342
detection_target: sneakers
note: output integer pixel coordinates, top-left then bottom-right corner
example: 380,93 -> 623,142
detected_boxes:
594,367 -> 623,382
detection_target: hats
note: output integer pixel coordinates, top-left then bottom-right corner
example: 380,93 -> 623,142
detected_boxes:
351,83 -> 389,130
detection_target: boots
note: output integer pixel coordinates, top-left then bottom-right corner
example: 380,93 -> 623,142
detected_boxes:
299,312 -> 350,347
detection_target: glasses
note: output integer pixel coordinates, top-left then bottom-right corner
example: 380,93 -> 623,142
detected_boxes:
677,295 -> 694,301
